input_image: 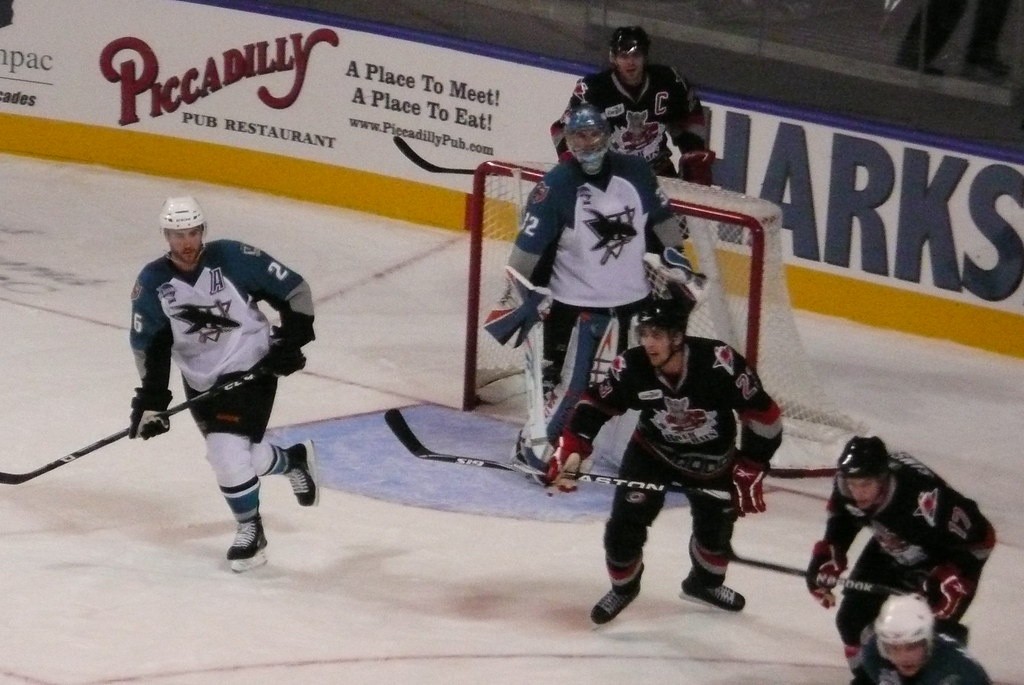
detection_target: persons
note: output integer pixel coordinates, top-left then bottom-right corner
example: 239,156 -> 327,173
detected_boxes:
888,0 -> 1012,90
485,109 -> 704,488
552,27 -> 727,187
807,436 -> 997,685
548,296 -> 783,626
128,196 -> 318,561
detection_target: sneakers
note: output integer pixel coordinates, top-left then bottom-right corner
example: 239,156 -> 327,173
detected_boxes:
591,564 -> 644,624
282,440 -> 319,507
227,513 -> 267,572
680,562 -> 746,613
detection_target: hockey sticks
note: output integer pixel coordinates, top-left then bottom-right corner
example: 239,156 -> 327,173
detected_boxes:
382,406 -> 741,502
697,507 -> 892,594
390,128 -> 506,177
0,369 -> 271,487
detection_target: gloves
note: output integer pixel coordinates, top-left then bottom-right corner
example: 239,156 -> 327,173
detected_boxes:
927,566 -> 968,623
679,152 -> 714,187
729,455 -> 769,515
265,327 -> 304,377
128,388 -> 173,439
545,426 -> 594,496
805,541 -> 846,608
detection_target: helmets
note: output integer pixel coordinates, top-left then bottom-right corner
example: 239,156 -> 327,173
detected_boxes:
568,107 -> 609,176
875,594 -> 934,660
160,195 -> 207,236
637,298 -> 689,333
612,26 -> 651,61
838,435 -> 889,478
